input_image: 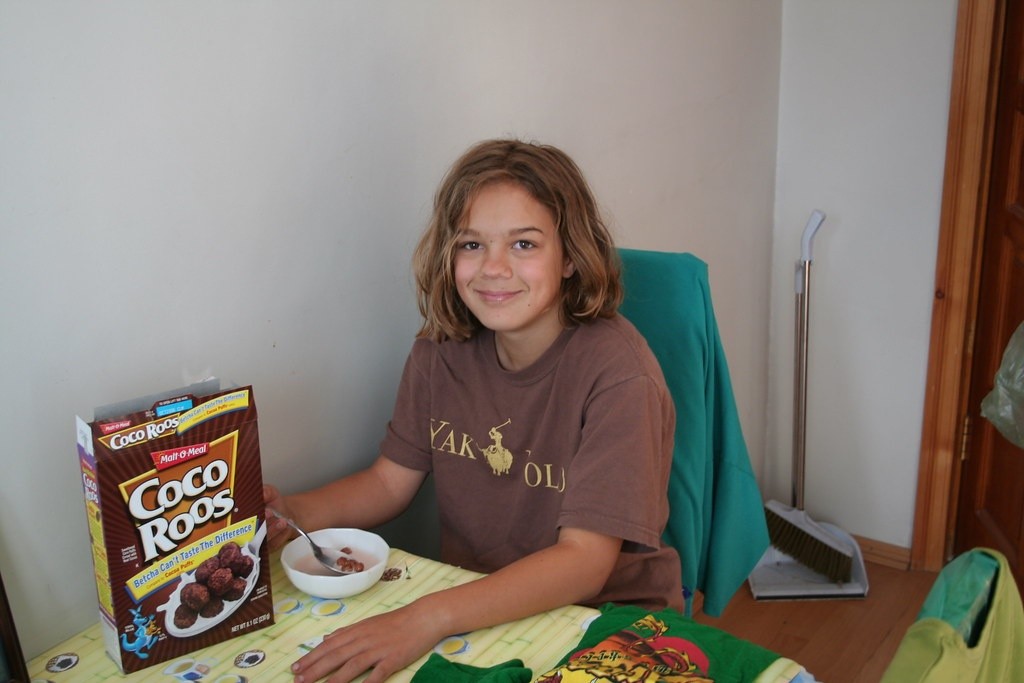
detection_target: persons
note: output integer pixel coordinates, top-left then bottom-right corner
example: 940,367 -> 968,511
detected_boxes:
261,138 -> 684,683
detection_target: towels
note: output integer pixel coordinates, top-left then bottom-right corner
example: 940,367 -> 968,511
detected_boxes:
613,251 -> 772,620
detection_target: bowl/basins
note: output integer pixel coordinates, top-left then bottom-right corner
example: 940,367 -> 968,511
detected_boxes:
282,528 -> 391,598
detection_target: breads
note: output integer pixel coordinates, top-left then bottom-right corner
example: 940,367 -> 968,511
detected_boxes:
174,541 -> 254,628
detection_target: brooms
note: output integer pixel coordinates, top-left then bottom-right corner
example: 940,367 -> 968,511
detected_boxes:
763,208 -> 854,585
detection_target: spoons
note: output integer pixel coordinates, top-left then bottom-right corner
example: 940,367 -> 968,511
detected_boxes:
264,504 -> 358,574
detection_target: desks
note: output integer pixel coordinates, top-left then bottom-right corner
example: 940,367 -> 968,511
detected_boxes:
23,540 -> 808,682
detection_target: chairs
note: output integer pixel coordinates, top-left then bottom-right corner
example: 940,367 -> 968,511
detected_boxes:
616,247 -> 771,618
881,547 -> 1024,683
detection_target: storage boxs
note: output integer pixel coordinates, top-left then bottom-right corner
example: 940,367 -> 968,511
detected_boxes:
76,377 -> 275,674
